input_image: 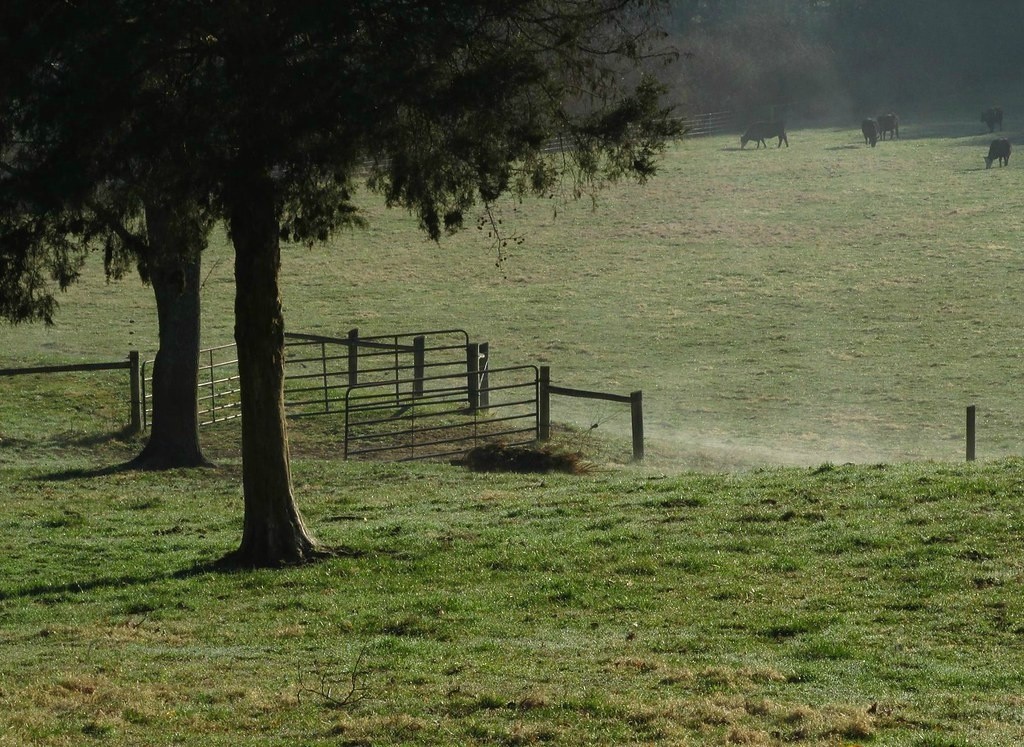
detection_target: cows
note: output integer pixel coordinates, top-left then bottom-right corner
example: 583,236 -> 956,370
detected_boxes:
740,122 -> 789,149
984,138 -> 1011,169
981,107 -> 1003,133
862,113 -> 899,148
